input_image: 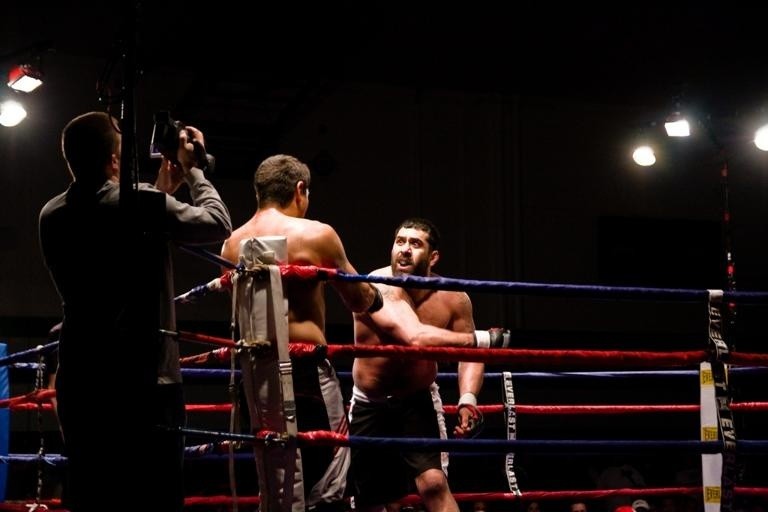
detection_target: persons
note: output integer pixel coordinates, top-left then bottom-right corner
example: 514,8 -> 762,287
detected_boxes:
346,214 -> 513,512
37,109 -> 235,511
391,493 -> 655,512
43,319 -> 64,447
218,152 -> 385,510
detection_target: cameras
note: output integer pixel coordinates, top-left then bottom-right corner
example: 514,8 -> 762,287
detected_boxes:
150,111 -> 216,173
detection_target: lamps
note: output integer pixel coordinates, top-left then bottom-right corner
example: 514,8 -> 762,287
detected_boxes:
0,63 -> 46,129
627,103 -> 768,353
